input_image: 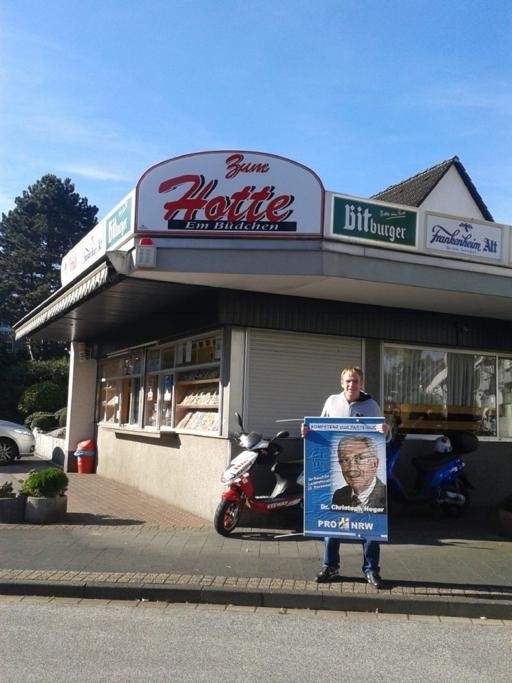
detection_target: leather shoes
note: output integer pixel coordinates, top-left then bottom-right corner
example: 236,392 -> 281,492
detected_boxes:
366,571 -> 382,588
315,567 -> 339,582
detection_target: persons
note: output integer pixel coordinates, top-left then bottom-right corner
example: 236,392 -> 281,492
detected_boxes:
298,365 -> 393,589
332,435 -> 385,513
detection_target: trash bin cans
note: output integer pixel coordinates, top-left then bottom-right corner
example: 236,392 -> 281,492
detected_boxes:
74,439 -> 96,474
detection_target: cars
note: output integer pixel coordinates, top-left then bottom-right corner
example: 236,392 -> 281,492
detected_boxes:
1,419 -> 35,465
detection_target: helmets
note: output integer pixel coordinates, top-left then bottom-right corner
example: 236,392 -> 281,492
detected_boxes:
434,437 -> 452,453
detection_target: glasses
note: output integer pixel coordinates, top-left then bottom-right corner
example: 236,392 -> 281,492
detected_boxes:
340,455 -> 374,465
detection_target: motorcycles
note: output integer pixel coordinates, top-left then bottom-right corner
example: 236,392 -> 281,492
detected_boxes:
386,413 -> 478,516
214,413 -> 303,535
